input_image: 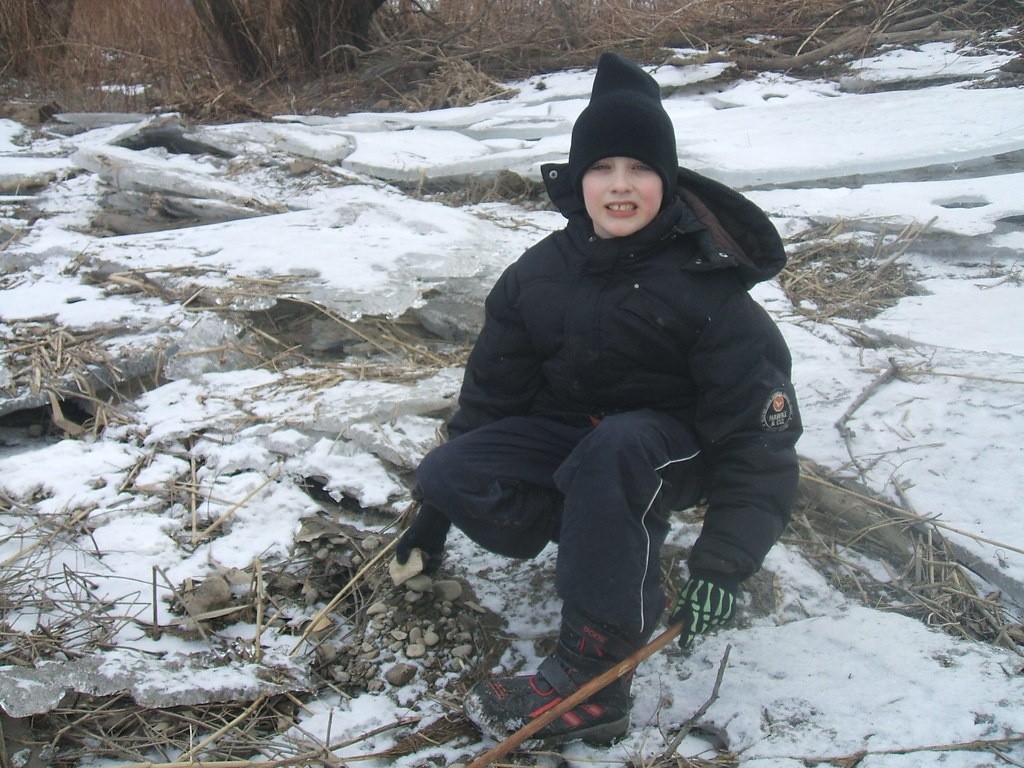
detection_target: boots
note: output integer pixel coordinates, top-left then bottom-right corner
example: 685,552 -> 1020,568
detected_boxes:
464,594 -> 669,752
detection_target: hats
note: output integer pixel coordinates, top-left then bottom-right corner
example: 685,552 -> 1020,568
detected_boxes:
568,52 -> 678,197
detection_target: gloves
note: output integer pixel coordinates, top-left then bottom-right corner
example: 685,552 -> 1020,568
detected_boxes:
396,505 -> 452,575
668,574 -> 737,650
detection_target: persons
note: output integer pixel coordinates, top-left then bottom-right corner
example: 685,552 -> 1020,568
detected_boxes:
396,52 -> 804,752
